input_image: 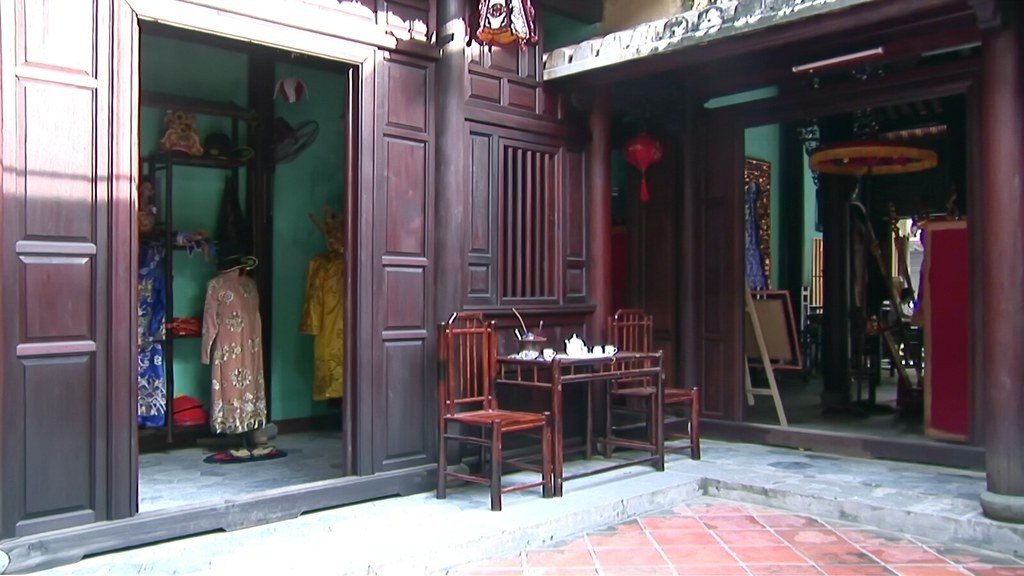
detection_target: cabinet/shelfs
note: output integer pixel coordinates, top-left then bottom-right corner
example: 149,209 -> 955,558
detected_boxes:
148,116 -> 256,446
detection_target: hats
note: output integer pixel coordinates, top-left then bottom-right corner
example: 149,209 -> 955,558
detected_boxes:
216,242 -> 258,273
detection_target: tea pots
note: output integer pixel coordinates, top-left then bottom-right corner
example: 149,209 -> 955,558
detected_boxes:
565,333 -> 585,356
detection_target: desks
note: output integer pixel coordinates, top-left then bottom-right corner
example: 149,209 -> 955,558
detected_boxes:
484,351 -> 667,498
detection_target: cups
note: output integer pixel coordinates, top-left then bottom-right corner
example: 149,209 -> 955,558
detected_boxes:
593,345 -> 618,356
518,348 -> 557,362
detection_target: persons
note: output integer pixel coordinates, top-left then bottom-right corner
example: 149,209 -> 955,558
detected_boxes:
890,287 -> 917,363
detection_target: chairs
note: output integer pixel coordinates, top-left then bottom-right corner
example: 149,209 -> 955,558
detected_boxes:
605,309 -> 700,468
878,300 -> 925,376
437,312 -> 554,511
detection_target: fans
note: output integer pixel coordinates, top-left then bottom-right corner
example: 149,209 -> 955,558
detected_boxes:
260,116 -> 320,166
